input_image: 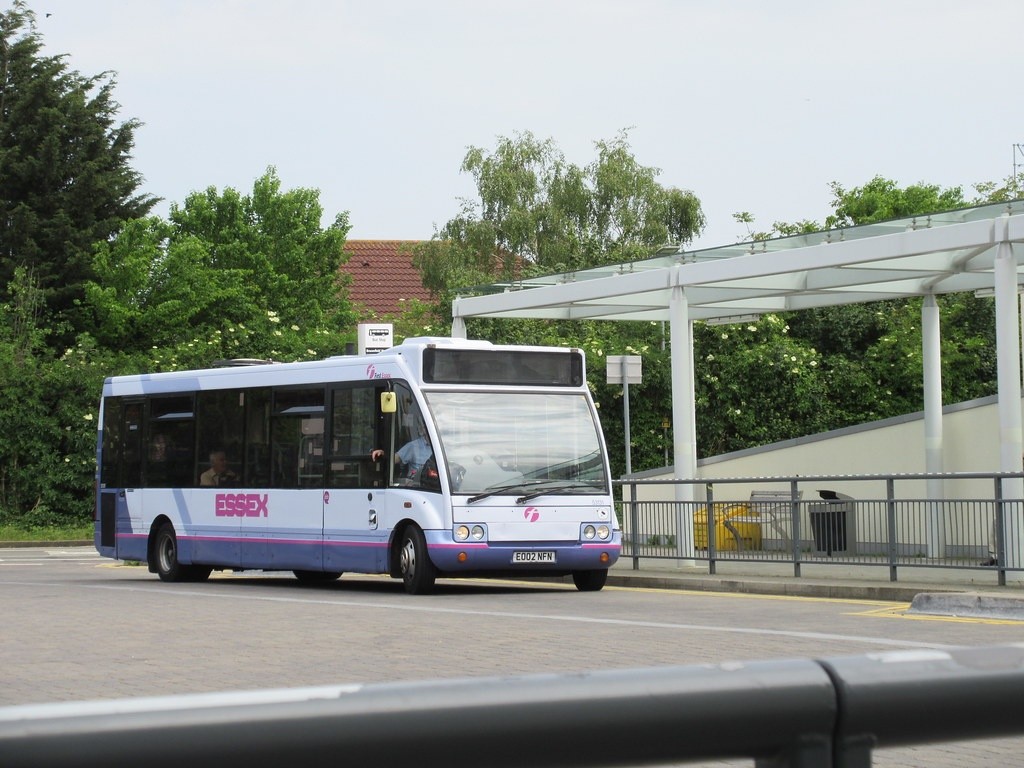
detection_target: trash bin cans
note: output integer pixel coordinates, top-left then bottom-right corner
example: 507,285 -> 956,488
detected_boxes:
807,489 -> 856,557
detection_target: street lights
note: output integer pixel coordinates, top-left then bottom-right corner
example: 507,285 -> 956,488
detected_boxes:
656,246 -> 680,466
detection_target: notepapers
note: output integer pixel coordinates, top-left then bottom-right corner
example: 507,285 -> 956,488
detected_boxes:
693,505 -> 762,552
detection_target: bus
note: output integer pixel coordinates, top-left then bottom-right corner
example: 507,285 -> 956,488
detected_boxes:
96,336 -> 620,595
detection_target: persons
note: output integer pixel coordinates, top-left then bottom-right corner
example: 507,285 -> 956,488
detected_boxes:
251,446 -> 282,488
199,450 -> 241,486
372,417 -> 433,479
140,434 -> 181,488
